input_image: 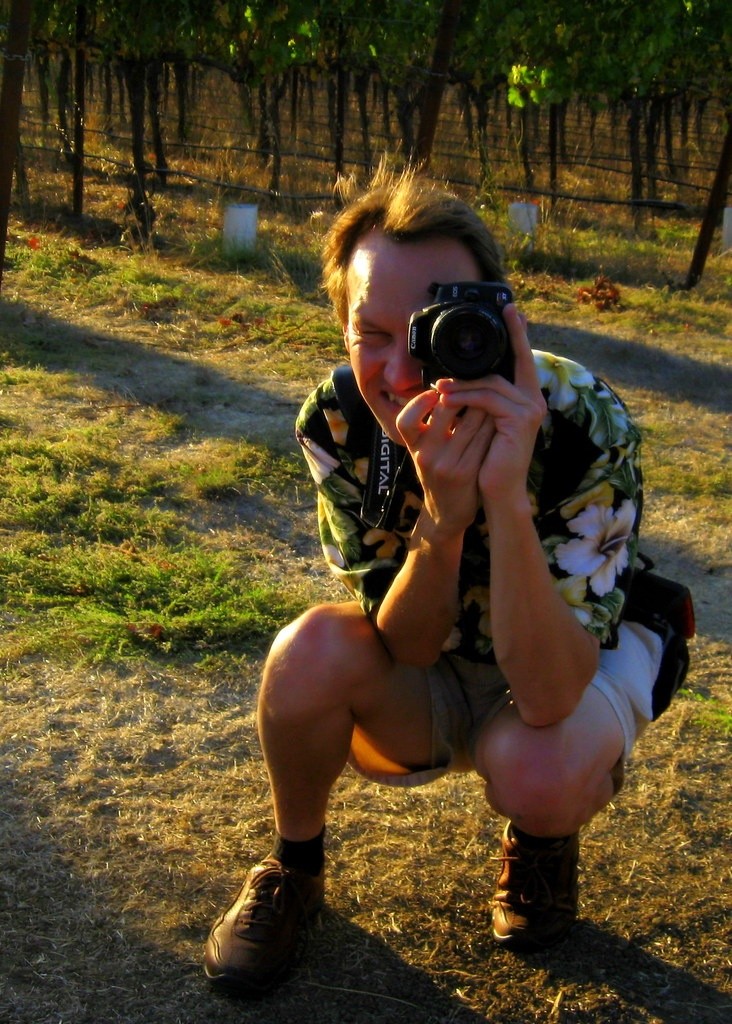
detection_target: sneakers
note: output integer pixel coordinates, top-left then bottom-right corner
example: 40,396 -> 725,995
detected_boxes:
203,848 -> 324,992
490,822 -> 579,949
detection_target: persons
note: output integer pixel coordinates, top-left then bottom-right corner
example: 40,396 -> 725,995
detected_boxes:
201,145 -> 696,999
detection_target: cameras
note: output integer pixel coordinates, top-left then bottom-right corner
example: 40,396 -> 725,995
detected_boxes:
408,280 -> 515,419
